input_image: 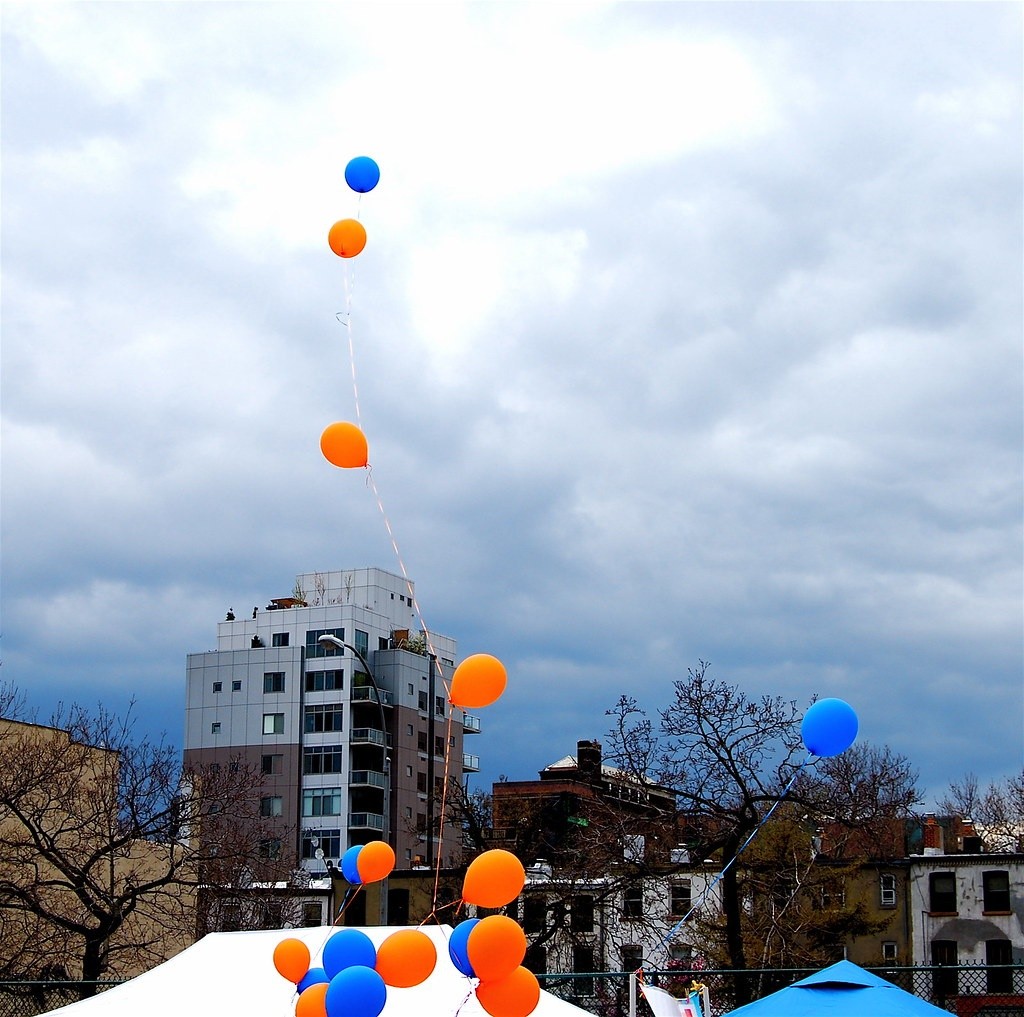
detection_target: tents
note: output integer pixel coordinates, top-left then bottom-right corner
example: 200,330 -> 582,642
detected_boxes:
39,924 -> 601,1017
720,959 -> 958,1017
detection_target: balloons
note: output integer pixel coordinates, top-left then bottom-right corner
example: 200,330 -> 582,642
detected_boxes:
328,219 -> 366,258
800,697 -> 859,757
447,652 -> 508,708
345,156 -> 380,193
320,420 -> 368,469
272,840 -> 541,1017
